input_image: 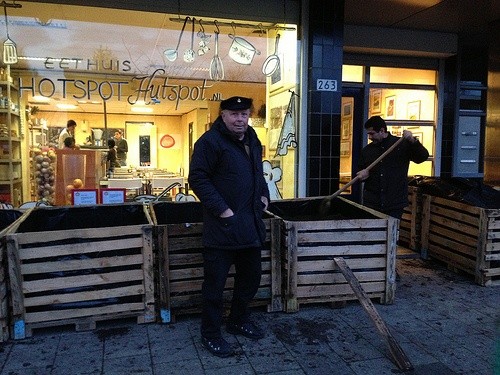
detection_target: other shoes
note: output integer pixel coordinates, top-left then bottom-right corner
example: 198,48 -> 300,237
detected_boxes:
396,272 -> 400,281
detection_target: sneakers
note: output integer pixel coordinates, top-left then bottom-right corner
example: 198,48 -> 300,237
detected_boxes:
226,322 -> 265,340
201,335 -> 234,357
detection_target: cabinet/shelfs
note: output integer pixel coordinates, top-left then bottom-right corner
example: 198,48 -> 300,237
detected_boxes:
0,73 -> 27,211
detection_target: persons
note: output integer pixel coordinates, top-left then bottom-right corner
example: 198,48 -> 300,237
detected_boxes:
105,139 -> 121,175
187,96 -> 270,359
355,115 -> 430,219
59,119 -> 77,150
62,137 -> 79,150
113,129 -> 128,166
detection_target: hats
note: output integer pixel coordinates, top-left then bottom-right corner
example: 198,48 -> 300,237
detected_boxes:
220,96 -> 253,110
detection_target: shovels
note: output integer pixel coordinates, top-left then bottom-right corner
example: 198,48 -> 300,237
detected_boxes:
315,135 -> 406,218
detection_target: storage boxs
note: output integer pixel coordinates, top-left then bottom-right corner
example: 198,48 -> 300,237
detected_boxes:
149,199 -> 286,324
398,184 -> 422,252
0,209 -> 62,353
424,193 -> 500,287
267,195 -> 400,313
6,205 -> 157,339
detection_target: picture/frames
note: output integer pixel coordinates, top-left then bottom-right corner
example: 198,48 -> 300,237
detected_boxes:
342,102 -> 352,120
412,132 -> 423,145
369,87 -> 382,113
406,101 -> 421,129
390,125 -> 403,138
385,95 -> 397,120
342,120 -> 349,140
339,141 -> 351,158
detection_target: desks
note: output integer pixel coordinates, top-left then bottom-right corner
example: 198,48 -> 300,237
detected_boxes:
108,179 -> 142,198
146,178 -> 184,196
152,174 -> 176,194
107,174 -> 133,179
55,148 -> 104,206
